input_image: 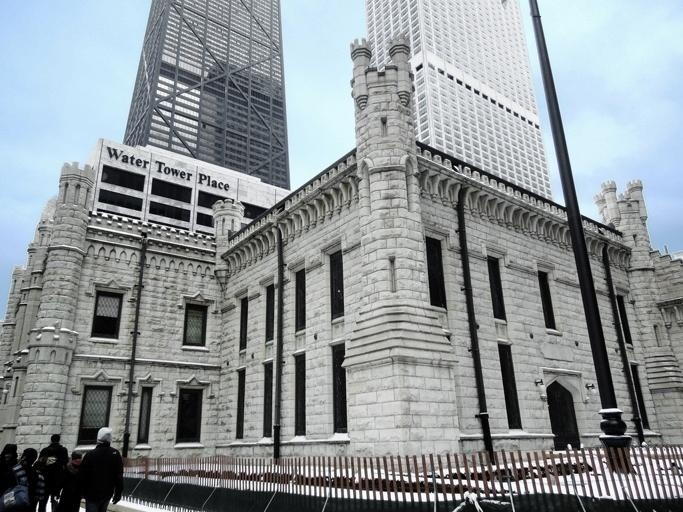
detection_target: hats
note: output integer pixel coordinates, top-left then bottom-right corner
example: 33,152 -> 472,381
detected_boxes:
1,444 -> 18,458
97,427 -> 113,444
24,448 -> 37,464
72,451 -> 82,460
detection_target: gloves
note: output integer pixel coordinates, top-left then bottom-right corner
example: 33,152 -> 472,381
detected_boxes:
112,493 -> 121,504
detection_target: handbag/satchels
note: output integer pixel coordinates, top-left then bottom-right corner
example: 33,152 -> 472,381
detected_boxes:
0,484 -> 29,512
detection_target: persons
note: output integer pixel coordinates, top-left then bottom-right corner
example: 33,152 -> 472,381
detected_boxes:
0,443 -> 17,468
35,434 -> 69,512
0,448 -> 40,512
77,427 -> 123,511
56,450 -> 82,512
